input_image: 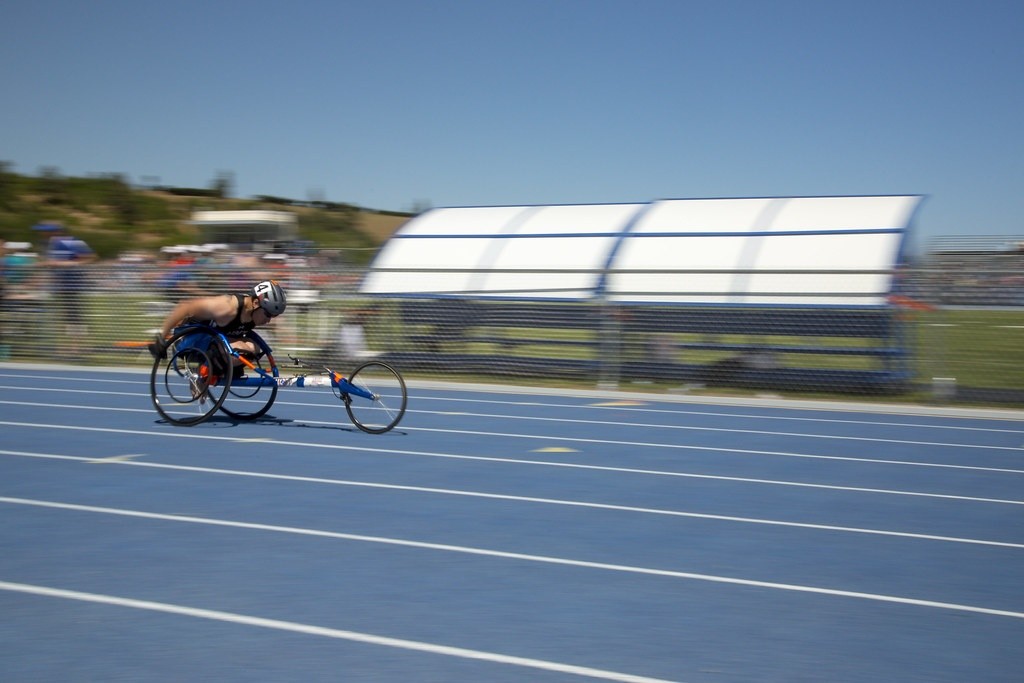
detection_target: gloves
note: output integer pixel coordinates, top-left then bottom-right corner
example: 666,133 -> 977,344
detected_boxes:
148,334 -> 168,360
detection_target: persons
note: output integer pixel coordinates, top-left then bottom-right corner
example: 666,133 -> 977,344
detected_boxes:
46,226 -> 99,335
148,279 -> 286,382
0,241 -> 39,361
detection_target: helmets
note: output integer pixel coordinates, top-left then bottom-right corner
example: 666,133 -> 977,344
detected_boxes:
253,280 -> 286,316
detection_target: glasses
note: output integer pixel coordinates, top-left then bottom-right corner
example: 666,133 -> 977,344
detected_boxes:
263,310 -> 278,319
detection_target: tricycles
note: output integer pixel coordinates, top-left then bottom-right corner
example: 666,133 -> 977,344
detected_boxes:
144,327 -> 408,435
